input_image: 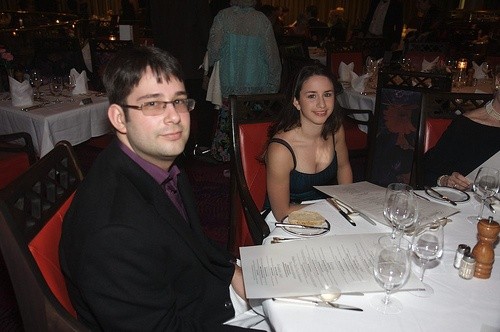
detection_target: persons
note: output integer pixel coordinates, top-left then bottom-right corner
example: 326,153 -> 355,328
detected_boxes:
117,0 -> 500,220
57,47 -> 269,332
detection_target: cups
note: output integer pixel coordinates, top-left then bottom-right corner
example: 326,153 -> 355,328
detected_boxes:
367,53 -> 500,90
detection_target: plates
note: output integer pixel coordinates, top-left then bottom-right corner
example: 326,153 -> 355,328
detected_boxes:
426,187 -> 470,203
280,215 -> 331,238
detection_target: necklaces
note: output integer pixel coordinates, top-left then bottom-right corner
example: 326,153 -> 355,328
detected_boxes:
485,100 -> 500,121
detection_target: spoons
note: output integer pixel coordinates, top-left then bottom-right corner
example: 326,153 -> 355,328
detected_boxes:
334,199 -> 359,216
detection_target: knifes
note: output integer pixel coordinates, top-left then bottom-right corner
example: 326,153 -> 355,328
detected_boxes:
336,199 -> 376,226
275,223 -> 330,231
272,297 -> 363,312
326,198 -> 356,227
425,185 -> 457,206
22,103 -> 49,112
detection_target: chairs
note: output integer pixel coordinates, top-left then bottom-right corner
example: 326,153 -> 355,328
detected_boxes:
0,40 -> 495,332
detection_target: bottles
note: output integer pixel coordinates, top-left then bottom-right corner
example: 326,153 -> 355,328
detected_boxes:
454,244 -> 476,280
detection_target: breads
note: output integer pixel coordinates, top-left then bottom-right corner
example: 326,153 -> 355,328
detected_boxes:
288,210 -> 324,225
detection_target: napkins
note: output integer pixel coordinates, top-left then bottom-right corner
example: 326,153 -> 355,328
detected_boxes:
338,60 -> 354,81
419,56 -> 439,80
7,75 -> 33,108
70,68 -> 89,95
472,61 -> 488,80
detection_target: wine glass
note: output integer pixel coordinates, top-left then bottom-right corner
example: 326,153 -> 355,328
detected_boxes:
29,71 -> 76,102
373,183 -> 444,312
466,168 -> 500,224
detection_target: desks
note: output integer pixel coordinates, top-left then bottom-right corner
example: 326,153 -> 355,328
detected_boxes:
336,74 -> 496,134
0,87 -> 110,159
262,190 -> 500,332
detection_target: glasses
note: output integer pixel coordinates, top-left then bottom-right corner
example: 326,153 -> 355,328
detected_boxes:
121,99 -> 196,116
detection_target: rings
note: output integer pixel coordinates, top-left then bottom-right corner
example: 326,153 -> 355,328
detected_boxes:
454,184 -> 457,188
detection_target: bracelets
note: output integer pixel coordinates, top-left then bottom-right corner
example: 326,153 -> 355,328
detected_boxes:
436,175 -> 448,187
